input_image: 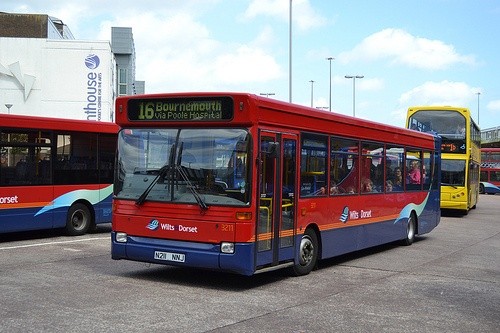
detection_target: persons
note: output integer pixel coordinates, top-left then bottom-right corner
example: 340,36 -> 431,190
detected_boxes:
412,124 -> 416,130
320,156 -> 425,194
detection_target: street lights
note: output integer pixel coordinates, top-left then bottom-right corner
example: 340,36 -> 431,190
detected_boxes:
309,80 -> 316,107
260,93 -> 275,98
476,92 -> 480,125
344,75 -> 365,117
5,103 -> 13,113
326,57 -> 334,111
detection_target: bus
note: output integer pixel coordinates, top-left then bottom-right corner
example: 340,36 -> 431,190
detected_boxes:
0,113 -> 122,238
480,147 -> 500,194
110,90 -> 442,278
404,104 -> 482,215
